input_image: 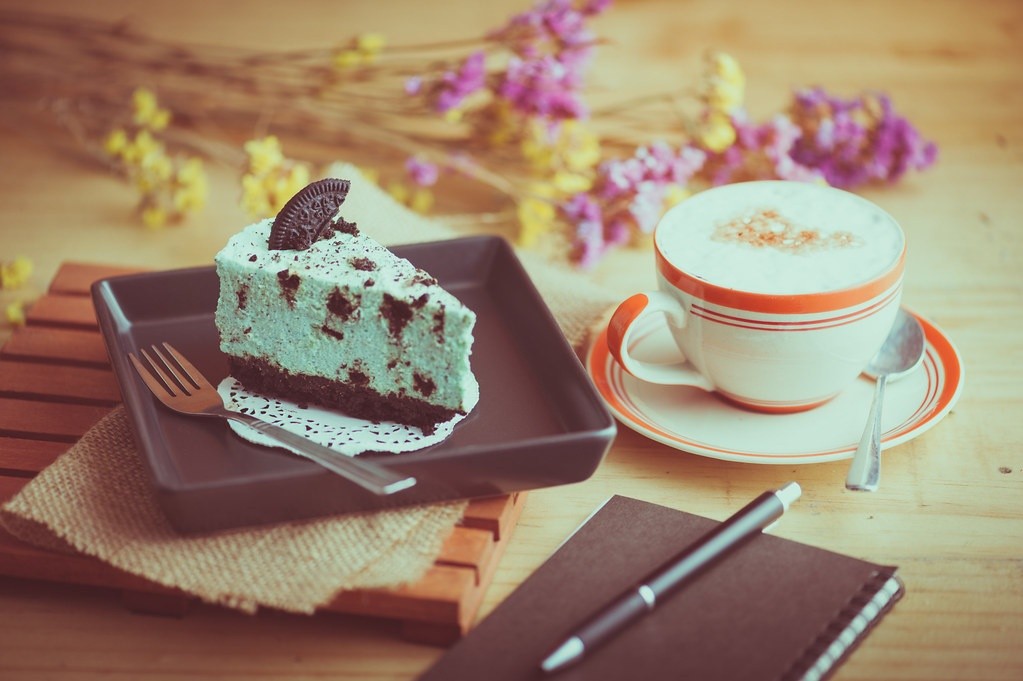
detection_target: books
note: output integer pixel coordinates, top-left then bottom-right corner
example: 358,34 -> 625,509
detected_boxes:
413,495 -> 906,681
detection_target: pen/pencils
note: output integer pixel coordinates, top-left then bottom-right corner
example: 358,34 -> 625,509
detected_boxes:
539,481 -> 804,675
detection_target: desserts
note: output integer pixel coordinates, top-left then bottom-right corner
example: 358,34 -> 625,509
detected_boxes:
212,176 -> 481,440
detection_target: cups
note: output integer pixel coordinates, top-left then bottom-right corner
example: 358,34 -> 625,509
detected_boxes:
605,176 -> 910,415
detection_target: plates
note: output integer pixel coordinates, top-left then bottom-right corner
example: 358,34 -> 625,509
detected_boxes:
89,233 -> 620,538
587,291 -> 966,466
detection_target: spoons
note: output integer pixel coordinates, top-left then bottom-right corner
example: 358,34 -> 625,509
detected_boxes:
845,304 -> 927,496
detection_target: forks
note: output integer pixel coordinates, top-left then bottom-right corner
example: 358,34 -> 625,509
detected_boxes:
125,339 -> 419,497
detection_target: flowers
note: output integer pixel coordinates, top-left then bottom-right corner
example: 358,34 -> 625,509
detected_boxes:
0,0 -> 941,344
